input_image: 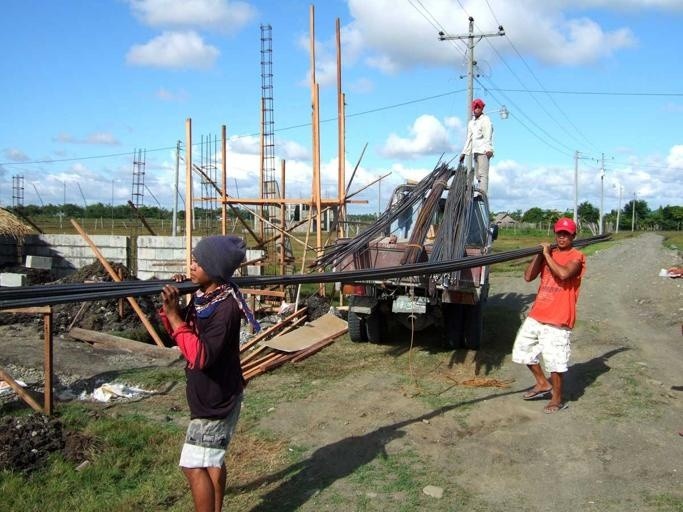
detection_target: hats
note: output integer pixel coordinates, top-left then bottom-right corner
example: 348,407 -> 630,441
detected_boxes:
555,218 -> 576,234
193,236 -> 246,283
472,100 -> 484,110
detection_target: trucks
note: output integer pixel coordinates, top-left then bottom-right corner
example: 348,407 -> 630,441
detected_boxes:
334,182 -> 498,348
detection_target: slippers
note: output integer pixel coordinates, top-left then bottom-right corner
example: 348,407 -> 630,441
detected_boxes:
544,402 -> 568,414
523,388 -> 551,399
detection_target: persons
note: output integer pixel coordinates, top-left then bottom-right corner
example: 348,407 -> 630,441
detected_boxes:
459,98 -> 493,193
159,235 -> 246,510
510,218 -> 586,414
